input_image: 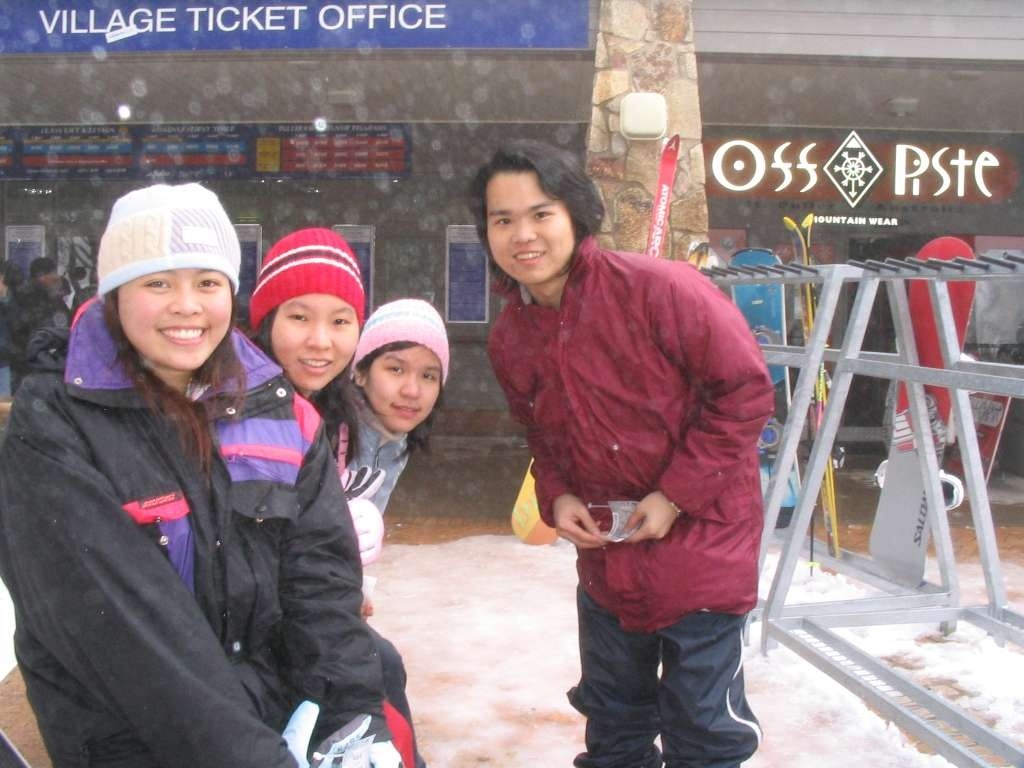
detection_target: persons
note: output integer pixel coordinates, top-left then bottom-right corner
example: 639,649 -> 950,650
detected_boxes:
0,183 -> 449,768
468,139 -> 762,767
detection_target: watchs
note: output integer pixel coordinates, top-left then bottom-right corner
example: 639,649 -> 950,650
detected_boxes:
664,497 -> 685,520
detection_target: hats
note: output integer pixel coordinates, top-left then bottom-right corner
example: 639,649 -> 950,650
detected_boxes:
248,226 -> 366,332
352,299 -> 450,387
96,182 -> 242,304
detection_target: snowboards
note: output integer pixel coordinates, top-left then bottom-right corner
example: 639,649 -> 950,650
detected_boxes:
943,359 -> 1011,498
864,237 -> 978,587
725,246 -> 801,528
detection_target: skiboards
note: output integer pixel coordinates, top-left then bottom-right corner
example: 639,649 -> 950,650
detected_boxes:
643,134 -> 680,258
781,213 -> 842,573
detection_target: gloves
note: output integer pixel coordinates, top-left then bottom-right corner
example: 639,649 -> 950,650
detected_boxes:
340,466 -> 386,499
346,498 -> 385,566
